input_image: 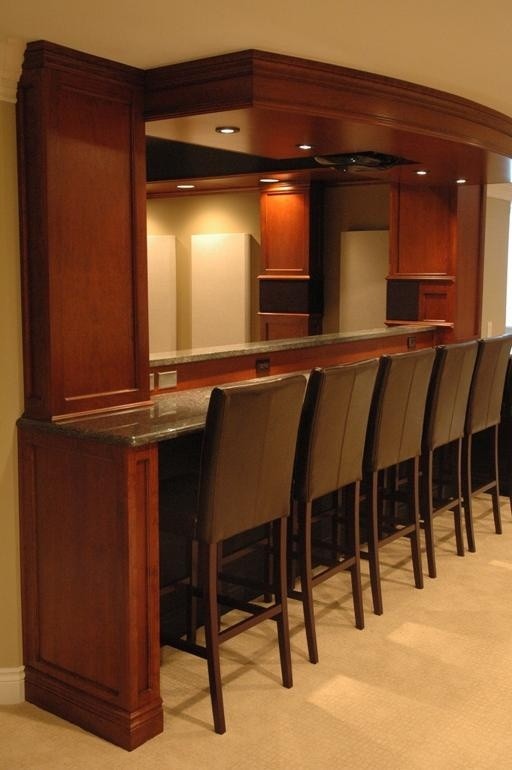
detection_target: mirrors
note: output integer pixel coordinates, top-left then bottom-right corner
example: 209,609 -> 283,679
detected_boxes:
146,135 -> 387,362
